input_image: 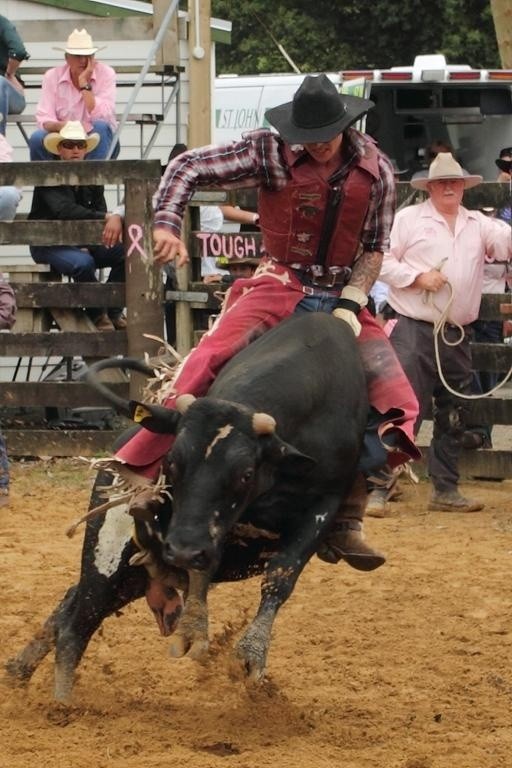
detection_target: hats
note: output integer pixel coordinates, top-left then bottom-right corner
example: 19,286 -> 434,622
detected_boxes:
410,152 -> 483,191
51,28 -> 109,57
265,73 -> 376,145
44,120 -> 101,155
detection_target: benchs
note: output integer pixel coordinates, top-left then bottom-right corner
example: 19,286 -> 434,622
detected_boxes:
0,35 -> 191,382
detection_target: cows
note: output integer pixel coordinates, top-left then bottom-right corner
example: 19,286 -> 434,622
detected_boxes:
5,311 -> 370,709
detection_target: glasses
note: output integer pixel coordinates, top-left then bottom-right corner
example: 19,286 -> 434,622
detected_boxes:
58,139 -> 88,150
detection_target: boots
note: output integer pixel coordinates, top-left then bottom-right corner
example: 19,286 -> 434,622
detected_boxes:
318,469 -> 385,571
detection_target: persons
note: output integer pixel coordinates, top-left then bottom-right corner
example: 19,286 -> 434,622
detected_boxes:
388,156 -> 408,182
413,142 -> 469,178
358,152 -> 512,517
28,28 -> 120,160
0,15 -> 29,135
116,74 -> 413,573
491,149 -> 511,224
0,132 -> 23,220
0,281 -> 14,495
26,121 -> 127,332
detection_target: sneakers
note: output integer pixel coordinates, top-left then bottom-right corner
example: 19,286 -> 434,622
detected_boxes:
365,483 -> 386,517
427,489 -> 485,511
